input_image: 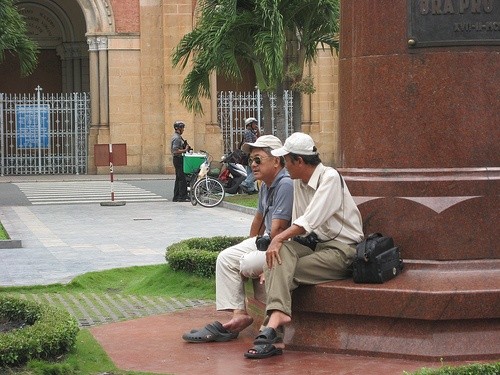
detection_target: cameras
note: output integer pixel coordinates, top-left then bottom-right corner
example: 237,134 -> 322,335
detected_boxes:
293,231 -> 318,251
255,236 -> 272,251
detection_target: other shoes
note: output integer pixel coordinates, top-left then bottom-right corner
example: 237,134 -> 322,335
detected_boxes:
179,198 -> 190,202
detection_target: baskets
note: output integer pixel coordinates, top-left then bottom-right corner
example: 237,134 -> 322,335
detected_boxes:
207,161 -> 223,177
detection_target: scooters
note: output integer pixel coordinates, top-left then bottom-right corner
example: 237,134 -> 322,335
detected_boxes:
187,138 -> 248,201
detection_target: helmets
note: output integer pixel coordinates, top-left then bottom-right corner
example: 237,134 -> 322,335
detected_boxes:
174,121 -> 185,129
246,118 -> 257,126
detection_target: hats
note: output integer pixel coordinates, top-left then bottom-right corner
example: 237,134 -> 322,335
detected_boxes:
241,135 -> 283,152
270,132 -> 318,156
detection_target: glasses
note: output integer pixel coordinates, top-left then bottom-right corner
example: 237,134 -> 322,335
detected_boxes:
248,156 -> 273,165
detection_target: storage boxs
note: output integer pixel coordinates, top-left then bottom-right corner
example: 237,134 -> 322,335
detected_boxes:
182,153 -> 207,173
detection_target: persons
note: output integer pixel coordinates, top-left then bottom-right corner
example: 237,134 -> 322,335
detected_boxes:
238,117 -> 261,194
246,132 -> 364,359
170,122 -> 189,202
215,134 -> 293,343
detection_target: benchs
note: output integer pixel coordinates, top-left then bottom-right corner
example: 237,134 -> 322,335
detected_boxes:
252,266 -> 500,321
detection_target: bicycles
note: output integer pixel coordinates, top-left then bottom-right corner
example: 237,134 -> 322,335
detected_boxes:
190,150 -> 225,208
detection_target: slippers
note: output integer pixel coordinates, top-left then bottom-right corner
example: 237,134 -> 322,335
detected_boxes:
244,344 -> 283,359
253,327 -> 282,344
182,320 -> 238,342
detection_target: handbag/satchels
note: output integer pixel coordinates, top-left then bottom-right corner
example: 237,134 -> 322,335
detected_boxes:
353,232 -> 403,284
